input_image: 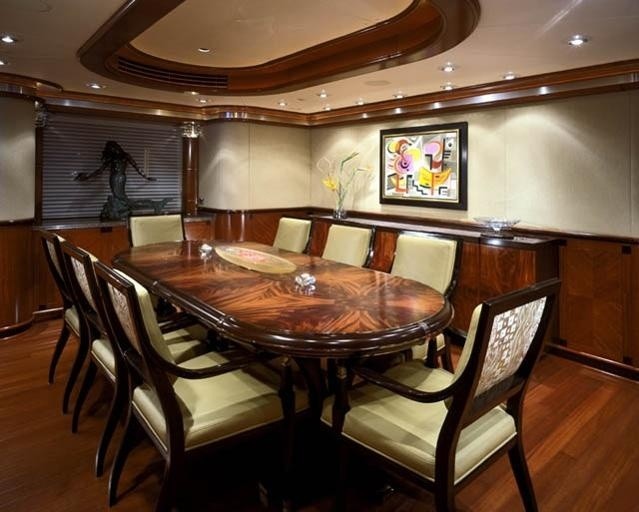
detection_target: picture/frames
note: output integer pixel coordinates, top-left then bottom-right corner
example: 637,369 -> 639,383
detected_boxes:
379,121 -> 468,211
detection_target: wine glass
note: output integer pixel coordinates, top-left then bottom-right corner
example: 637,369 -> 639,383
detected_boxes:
475,217 -> 521,238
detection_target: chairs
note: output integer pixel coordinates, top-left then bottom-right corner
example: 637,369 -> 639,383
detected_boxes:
319,276 -> 563,512
61,241 -> 230,477
40,228 -> 177,415
126,215 -> 186,248
273,217 -> 312,253
321,223 -> 377,267
91,261 -> 312,512
327,230 -> 464,396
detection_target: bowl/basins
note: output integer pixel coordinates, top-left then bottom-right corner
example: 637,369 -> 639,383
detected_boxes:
295,275 -> 318,289
197,248 -> 215,255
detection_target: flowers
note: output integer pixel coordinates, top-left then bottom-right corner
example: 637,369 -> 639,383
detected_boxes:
316,150 -> 374,212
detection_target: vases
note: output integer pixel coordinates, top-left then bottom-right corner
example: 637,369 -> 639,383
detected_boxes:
331,188 -> 347,219
